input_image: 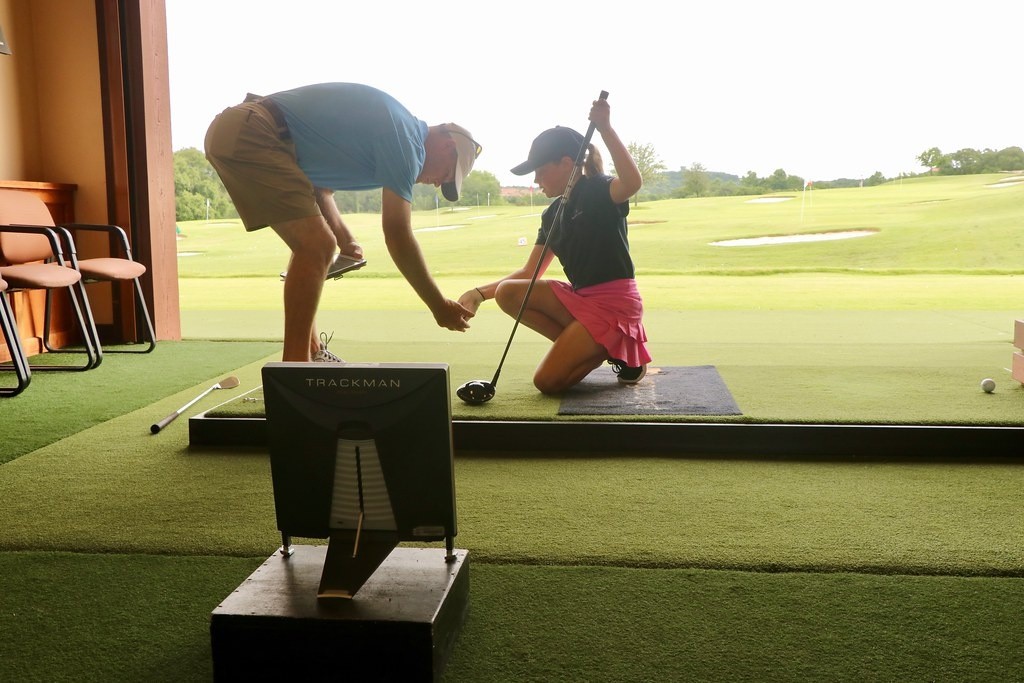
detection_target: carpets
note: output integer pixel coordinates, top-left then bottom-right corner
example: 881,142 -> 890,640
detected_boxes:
557,365 -> 743,414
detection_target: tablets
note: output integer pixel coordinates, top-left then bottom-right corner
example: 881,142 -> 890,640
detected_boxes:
280,253 -> 368,281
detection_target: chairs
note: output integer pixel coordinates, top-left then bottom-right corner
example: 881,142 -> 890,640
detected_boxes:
0,191 -> 157,398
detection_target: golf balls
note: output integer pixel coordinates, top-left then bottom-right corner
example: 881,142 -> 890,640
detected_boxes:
981,379 -> 995,392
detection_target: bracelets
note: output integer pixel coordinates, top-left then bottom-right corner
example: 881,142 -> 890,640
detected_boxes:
476,288 -> 485,300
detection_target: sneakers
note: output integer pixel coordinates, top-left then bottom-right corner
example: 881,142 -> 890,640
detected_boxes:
607,357 -> 647,383
312,330 -> 345,363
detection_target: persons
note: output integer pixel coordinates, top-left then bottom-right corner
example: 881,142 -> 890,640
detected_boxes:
204,82 -> 481,362
458,98 -> 653,396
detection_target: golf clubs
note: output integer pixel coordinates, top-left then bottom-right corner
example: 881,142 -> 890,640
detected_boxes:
150,376 -> 240,434
455,89 -> 610,405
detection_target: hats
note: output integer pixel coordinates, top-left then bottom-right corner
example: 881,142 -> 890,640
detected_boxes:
510,126 -> 584,176
441,123 -> 475,202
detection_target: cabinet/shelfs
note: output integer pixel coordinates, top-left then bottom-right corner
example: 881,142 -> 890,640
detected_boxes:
0,181 -> 78,366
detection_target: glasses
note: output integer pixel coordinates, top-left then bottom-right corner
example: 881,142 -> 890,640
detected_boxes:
441,130 -> 482,159
